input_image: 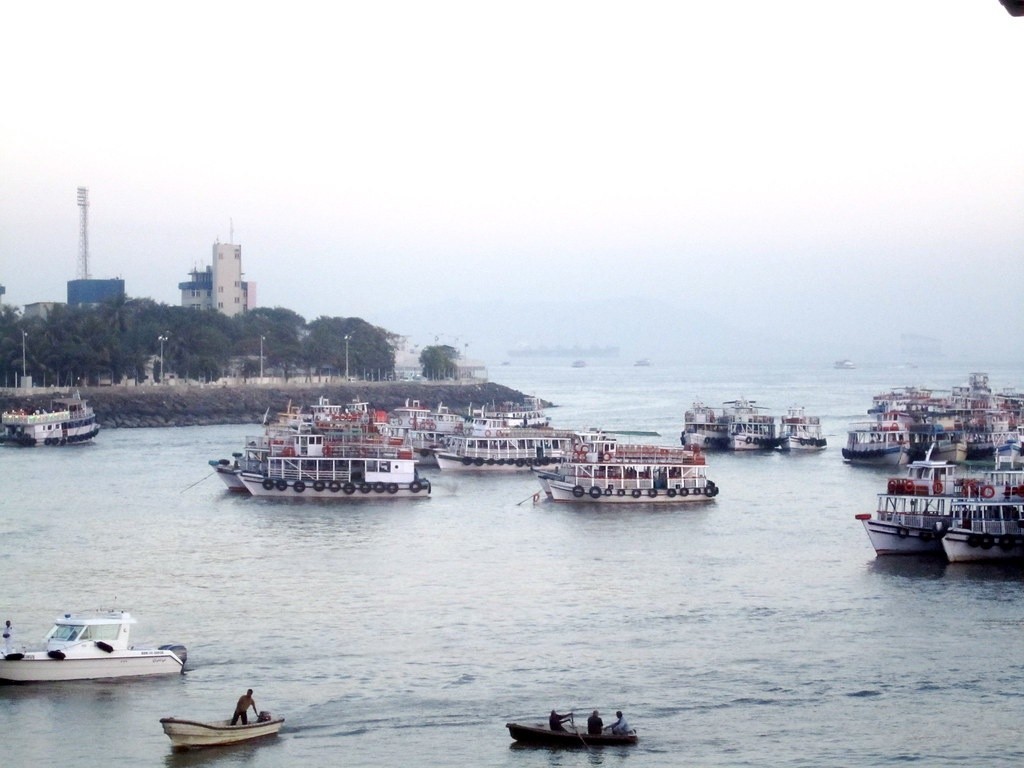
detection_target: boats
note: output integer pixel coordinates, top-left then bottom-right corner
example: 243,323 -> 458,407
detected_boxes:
778,405 -> 827,451
854,445 -> 1024,563
868,372 -> 1024,463
207,397 -> 431,498
681,401 -> 726,450
160,711 -> 285,747
0,390 -> 100,447
531,430 -> 720,501
505,721 -> 638,744
842,422 -> 911,466
725,400 -> 775,450
380,398 -> 606,472
0,596 -> 187,682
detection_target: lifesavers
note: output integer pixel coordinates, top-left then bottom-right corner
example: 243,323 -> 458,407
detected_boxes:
888,480 -> 943,493
485,430 -> 502,437
263,478 -> 423,494
800,438 -> 827,447
461,456 -> 566,467
333,414 -> 353,421
398,418 -> 437,430
763,416 -> 774,423
968,534 -> 1016,552
842,448 -> 886,460
573,444 -> 589,462
287,448 -> 295,456
898,519 -> 951,542
967,482 -> 994,498
322,447 -> 330,455
603,453 -> 611,461
746,437 -> 785,447
572,485 -> 715,498
454,427 -> 474,435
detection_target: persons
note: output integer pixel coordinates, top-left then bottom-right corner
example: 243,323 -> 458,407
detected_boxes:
3,621 -> 13,654
604,711 -> 632,735
588,710 -> 603,734
231,689 -> 260,725
549,710 -> 573,733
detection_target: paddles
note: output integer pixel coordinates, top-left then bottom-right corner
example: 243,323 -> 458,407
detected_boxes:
569,719 -> 590,750
